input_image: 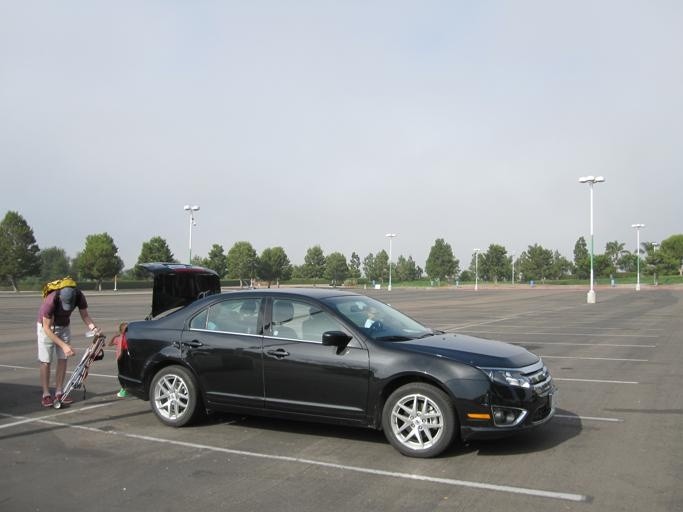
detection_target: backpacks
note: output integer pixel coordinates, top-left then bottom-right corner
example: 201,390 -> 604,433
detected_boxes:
42,276 -> 81,307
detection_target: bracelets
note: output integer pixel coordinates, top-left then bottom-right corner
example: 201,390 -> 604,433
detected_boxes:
91,327 -> 96,334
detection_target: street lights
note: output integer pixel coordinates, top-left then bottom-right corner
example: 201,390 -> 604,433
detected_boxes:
632,223 -> 646,290
650,242 -> 660,286
576,175 -> 602,305
474,248 -> 479,291
384,234 -> 395,292
184,203 -> 199,267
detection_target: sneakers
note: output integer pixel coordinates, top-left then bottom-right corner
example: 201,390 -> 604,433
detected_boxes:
42,394 -> 53,406
116,388 -> 128,397
54,394 -> 73,403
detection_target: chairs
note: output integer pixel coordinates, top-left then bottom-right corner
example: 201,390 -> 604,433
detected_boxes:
228,300 -> 336,341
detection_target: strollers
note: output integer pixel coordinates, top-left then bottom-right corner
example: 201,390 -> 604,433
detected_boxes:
51,334 -> 104,410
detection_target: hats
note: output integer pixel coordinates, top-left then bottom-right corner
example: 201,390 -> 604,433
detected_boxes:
61,287 -> 77,311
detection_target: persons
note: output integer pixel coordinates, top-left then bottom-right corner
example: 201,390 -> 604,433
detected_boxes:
36,285 -> 98,407
108,321 -> 130,399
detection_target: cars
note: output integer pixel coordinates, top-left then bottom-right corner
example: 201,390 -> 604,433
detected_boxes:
113,261 -> 556,460
